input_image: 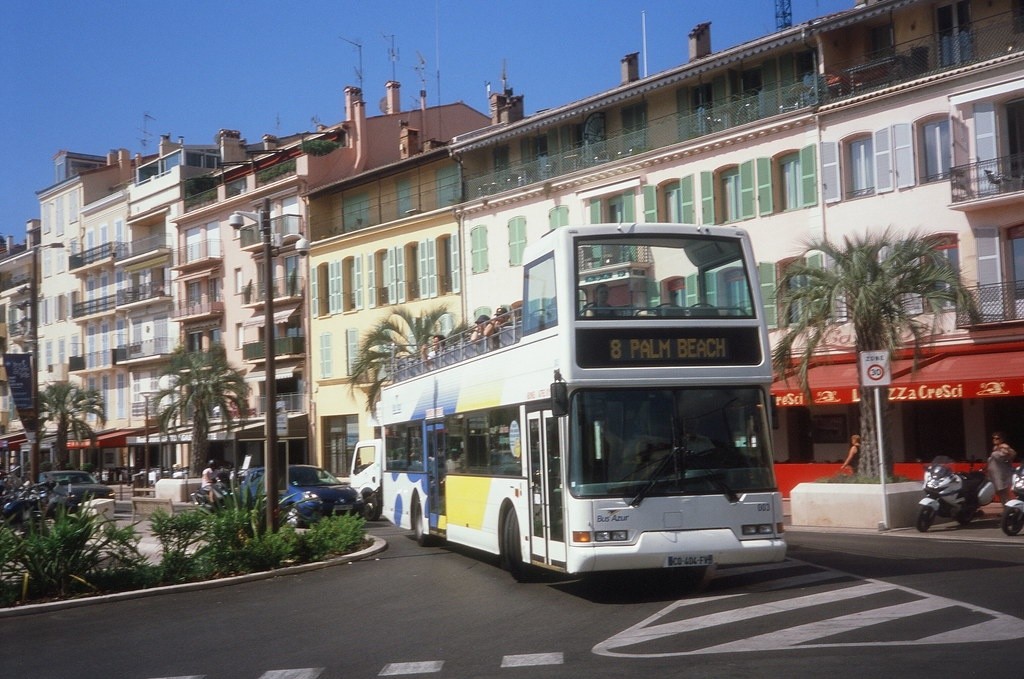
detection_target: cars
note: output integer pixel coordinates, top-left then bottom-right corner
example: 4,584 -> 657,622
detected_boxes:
94,467 -> 187,492
1,470 -> 114,525
227,464 -> 363,530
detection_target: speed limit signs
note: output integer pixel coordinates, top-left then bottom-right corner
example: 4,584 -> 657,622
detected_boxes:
860,347 -> 890,388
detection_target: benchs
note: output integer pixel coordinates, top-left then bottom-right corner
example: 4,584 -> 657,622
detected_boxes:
131,497 -> 187,528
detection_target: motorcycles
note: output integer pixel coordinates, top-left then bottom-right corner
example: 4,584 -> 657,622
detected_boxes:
913,455 -> 990,532
1001,461 -> 1024,537
193,468 -> 235,515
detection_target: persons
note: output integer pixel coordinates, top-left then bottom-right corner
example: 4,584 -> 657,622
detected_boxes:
426,335 -> 435,360
397,436 -> 435,472
841,435 -> 861,474
471,315 -> 490,354
446,448 -> 461,474
622,436 -> 652,481
422,335 -> 445,361
484,308 -> 513,350
988,432 -> 1016,505
202,460 -> 226,508
586,284 -> 614,317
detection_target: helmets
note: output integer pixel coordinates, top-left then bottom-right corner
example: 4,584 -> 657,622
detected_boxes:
208,460 -> 218,467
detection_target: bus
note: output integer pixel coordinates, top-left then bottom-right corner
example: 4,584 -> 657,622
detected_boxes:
383,222 -> 790,586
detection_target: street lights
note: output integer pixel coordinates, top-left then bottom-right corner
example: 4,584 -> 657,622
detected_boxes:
231,199 -> 310,536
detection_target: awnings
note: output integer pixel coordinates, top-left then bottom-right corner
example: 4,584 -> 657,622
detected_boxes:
770,342 -> 1024,407
126,414 -> 307,444
0,432 -> 26,451
66,426 -> 161,448
20,429 -> 116,451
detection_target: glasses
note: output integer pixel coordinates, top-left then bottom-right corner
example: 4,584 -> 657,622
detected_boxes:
992,437 -> 1001,440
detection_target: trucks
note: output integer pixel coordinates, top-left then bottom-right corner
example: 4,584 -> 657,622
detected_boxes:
349,440 -> 382,520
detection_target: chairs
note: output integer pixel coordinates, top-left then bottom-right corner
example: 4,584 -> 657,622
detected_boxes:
582,302 -> 722,317
